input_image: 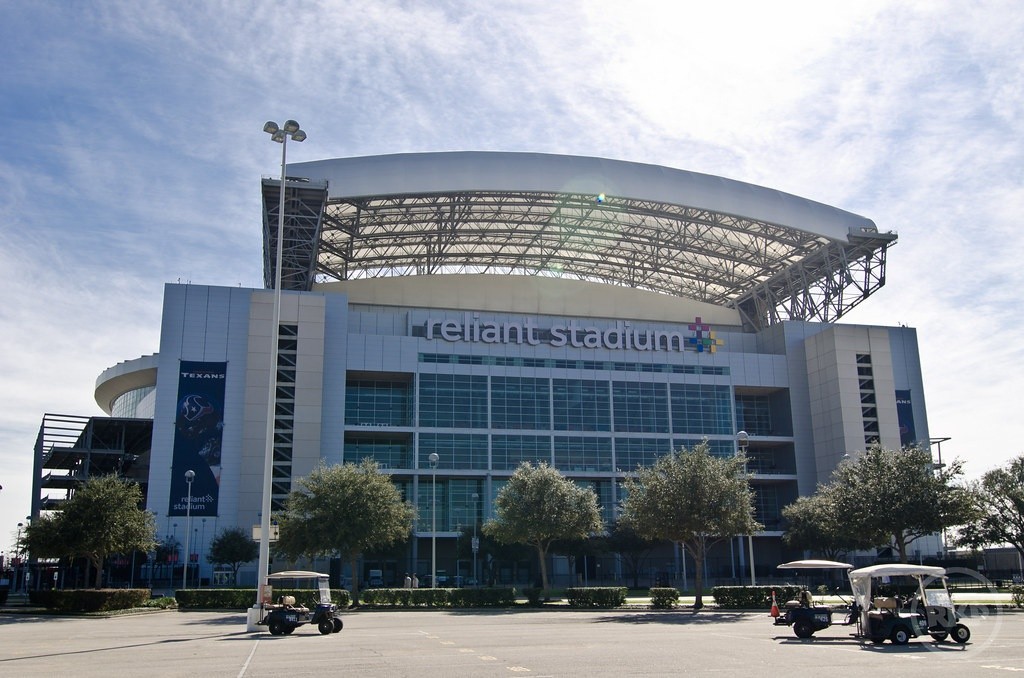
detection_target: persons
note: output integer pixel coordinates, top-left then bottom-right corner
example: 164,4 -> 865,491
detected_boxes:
413,573 -> 418,588
404,573 -> 411,588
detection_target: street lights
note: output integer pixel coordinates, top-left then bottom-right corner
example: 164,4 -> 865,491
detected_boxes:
244,115 -> 310,639
428,451 -> 440,588
178,467 -> 198,589
12,523 -> 23,594
0,568 -> 11,602
22,515 -> 35,594
471,493 -> 479,588
736,430 -> 757,587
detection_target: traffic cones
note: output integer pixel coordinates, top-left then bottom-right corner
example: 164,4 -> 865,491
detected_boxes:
768,590 -> 782,616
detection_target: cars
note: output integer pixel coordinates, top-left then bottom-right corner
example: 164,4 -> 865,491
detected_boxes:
467,576 -> 482,584
453,576 -> 464,587
339,575 -> 353,592
420,575 -> 439,587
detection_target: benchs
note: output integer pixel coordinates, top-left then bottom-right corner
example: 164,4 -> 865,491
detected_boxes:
868,596 -> 911,620
785,591 -> 831,611
265,594 -> 309,614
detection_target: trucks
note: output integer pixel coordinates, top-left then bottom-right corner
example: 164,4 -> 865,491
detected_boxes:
437,570 -> 449,586
368,569 -> 384,587
